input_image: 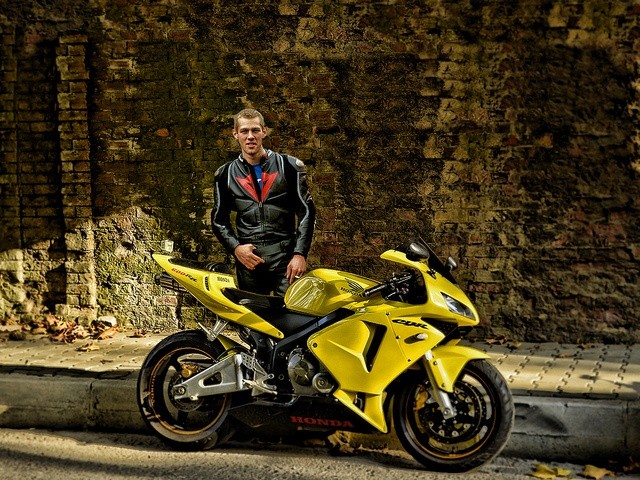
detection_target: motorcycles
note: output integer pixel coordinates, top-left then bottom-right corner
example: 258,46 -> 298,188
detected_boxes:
136,236 -> 515,473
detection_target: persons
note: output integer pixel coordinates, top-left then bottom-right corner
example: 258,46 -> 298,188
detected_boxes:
211,109 -> 316,297
